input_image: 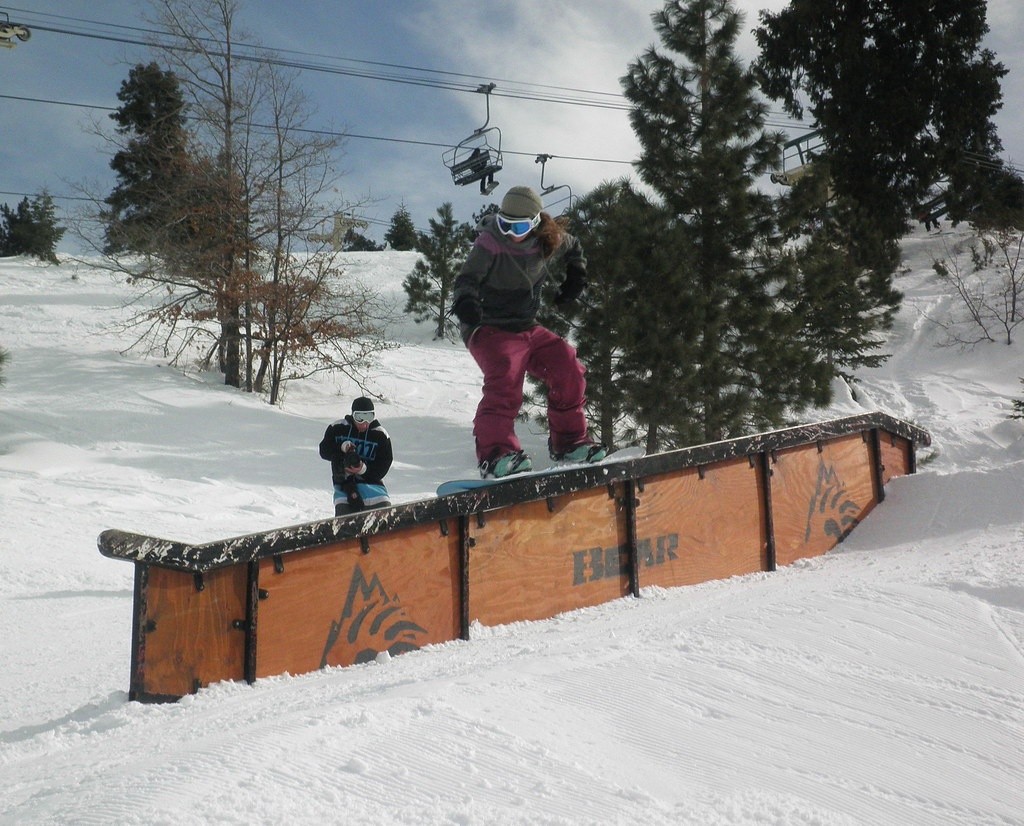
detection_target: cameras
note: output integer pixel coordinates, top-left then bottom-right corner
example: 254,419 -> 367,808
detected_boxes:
346,445 -> 361,469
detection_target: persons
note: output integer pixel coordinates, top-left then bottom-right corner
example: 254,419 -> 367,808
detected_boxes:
466,147 -> 496,193
452,185 -> 610,483
317,396 -> 395,519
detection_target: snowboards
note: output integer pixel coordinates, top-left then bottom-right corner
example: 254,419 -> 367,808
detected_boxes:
480,180 -> 499,196
429,441 -> 653,500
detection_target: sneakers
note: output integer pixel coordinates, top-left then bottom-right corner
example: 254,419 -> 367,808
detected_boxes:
555,444 -> 607,462
480,454 -> 533,479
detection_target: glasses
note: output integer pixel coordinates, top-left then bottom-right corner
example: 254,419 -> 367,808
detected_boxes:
496,214 -> 532,238
353,411 -> 376,424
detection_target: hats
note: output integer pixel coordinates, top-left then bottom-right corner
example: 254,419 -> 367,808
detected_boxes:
352,397 -> 374,410
501,185 -> 544,219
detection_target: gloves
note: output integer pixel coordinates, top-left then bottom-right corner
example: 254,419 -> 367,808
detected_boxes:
455,293 -> 483,326
559,267 -> 588,302
